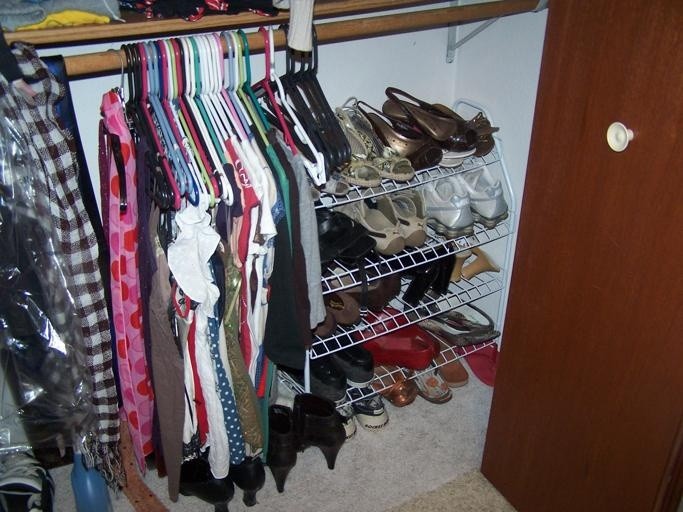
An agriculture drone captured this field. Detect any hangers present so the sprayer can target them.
[100,20,350,214]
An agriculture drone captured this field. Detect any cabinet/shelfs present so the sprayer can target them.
[277,100,527,412]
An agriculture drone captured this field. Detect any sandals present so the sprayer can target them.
[313,88,498,202]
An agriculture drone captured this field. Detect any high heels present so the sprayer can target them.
[180,394,346,512]
[279,250,499,340]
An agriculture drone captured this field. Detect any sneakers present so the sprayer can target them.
[304,297,500,440]
[314,162,508,270]
